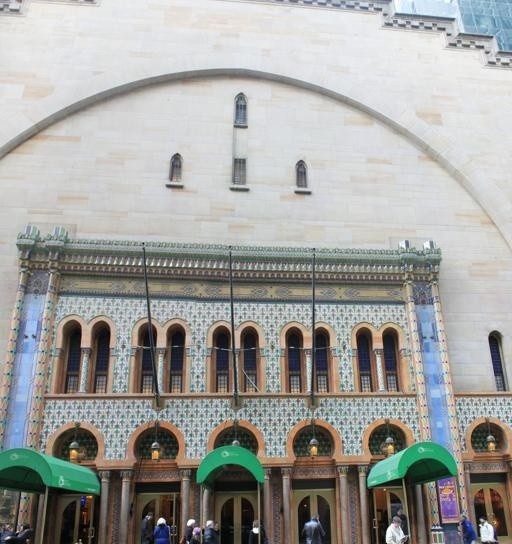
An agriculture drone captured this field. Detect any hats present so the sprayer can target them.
[157,518,166,526]
[187,519,213,534]
[393,517,401,522]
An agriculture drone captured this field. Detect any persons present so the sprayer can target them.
[140,512,155,543]
[459,514,498,544]
[153,517,169,544]
[0,520,30,544]
[183,518,219,544]
[249,520,266,544]
[386,516,409,544]
[301,513,326,544]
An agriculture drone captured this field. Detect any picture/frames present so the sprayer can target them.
[436,476,460,524]
